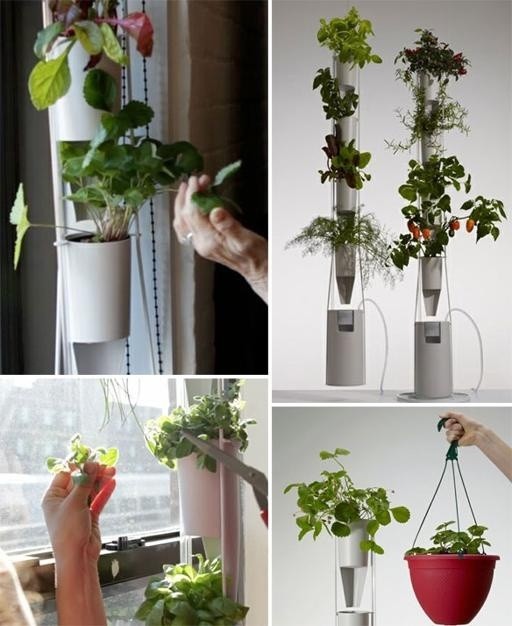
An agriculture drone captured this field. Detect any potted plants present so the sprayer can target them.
[284,448,410,569]
[144,379,257,539]
[9,101,244,345]
[28,0,154,143]
[404,520,500,626]
[283,6,508,316]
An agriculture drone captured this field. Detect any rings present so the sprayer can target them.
[185,230,198,243]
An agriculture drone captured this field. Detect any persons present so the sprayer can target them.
[41,452,116,626]
[172,173,266,308]
[436,411,512,482]
